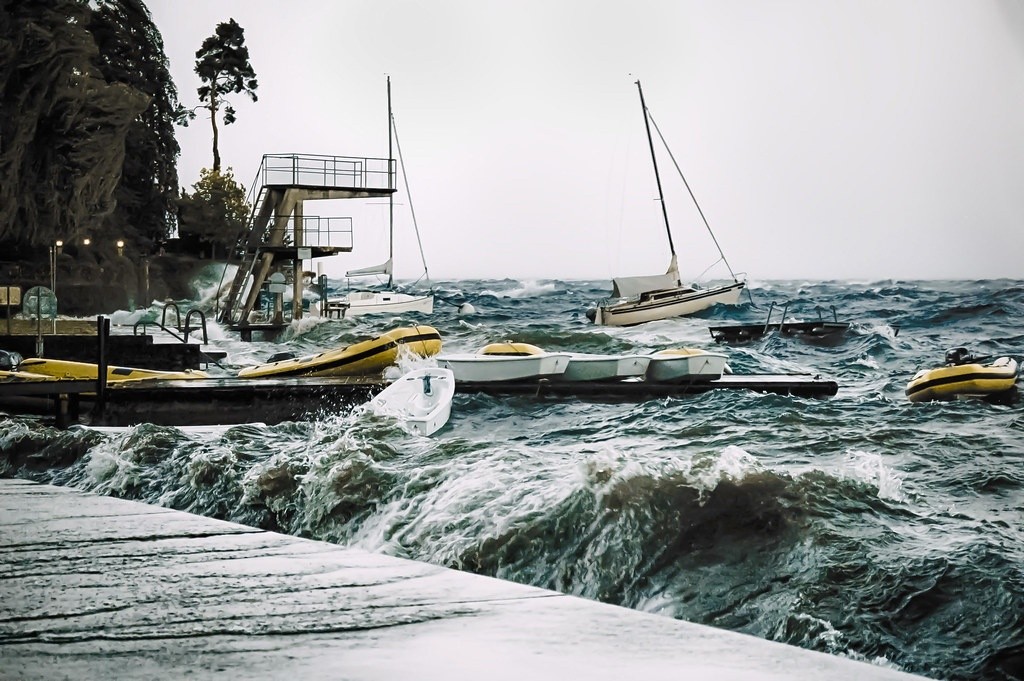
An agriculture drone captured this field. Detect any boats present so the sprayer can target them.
[0,350,58,383]
[19,358,212,382]
[707,299,900,346]
[644,346,729,382]
[239,320,444,381]
[476,342,546,356]
[434,348,573,383]
[905,346,1019,403]
[552,352,652,382]
[359,365,455,436]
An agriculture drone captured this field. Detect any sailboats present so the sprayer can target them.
[586,79,750,327]
[308,73,436,318]
